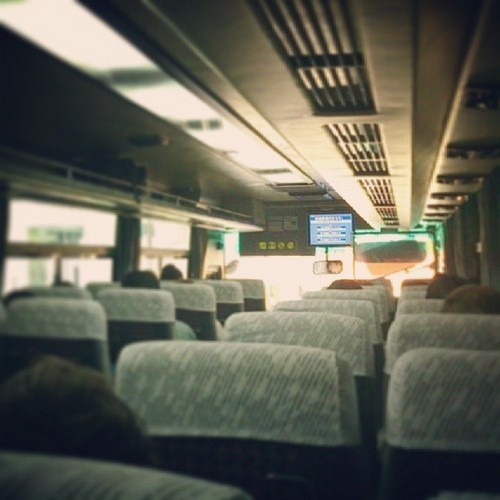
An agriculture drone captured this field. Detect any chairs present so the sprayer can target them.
[98,288,177,373]
[0,299,111,378]
[273,280,448,358]
[161,284,218,342]
[379,347,499,500]
[194,280,244,326]
[224,311,375,461]
[383,314,500,410]
[239,279,267,312]
[115,340,357,500]
[1,452,255,500]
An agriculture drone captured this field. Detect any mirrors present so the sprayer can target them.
[313,261,342,275]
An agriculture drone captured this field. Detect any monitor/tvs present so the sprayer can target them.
[308,212,355,248]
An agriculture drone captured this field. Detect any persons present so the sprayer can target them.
[0,352,145,468]
[328,271,500,316]
[0,265,237,351]
